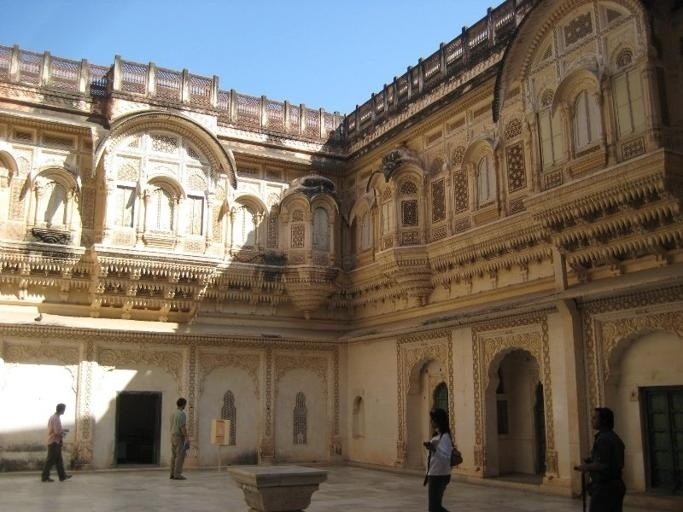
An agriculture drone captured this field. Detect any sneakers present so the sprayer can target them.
[169,475,186,480]
[42,473,73,483]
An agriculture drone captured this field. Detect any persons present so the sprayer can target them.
[423,407,453,512]
[169,398,190,480]
[41,403,73,482]
[574,407,626,512]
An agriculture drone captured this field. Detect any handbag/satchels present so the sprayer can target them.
[449,448,463,467]
